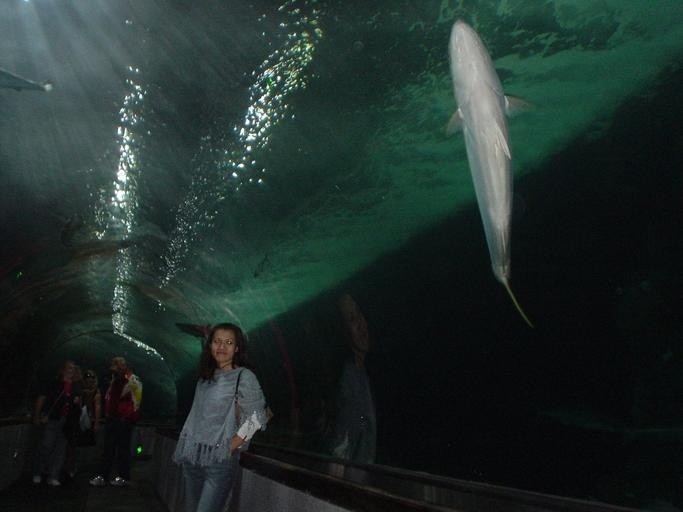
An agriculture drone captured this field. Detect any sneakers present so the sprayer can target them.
[32,472,129,487]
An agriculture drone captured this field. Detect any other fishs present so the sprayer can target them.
[442,19,538,328]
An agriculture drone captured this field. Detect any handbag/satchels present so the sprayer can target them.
[32,413,49,425]
[232,367,274,433]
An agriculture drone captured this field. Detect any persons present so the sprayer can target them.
[169,323,270,512]
[322,287,382,487]
[21,352,147,491]
